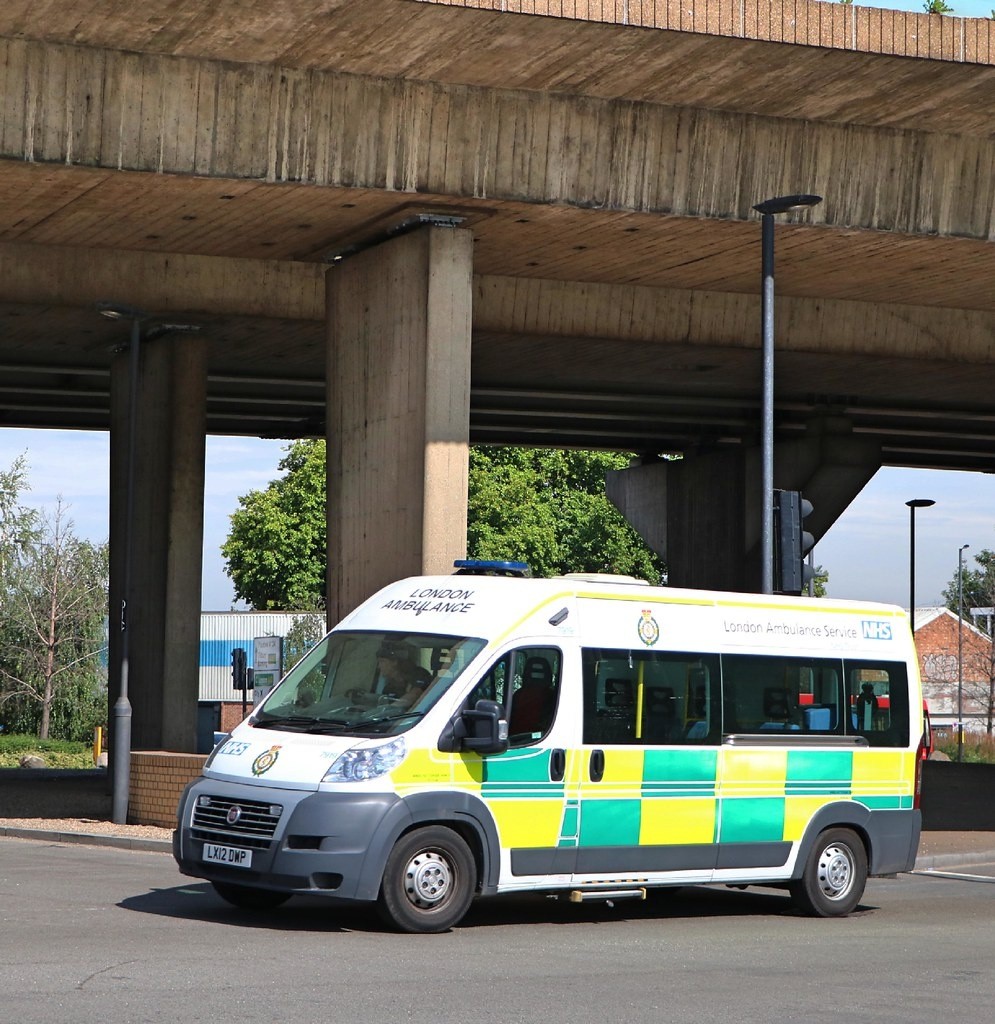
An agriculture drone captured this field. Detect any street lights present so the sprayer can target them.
[752,193,823,592]
[907,498,936,641]
[957,544,971,761]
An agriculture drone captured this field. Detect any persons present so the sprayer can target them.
[355,640,433,710]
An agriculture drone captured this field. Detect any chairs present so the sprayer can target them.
[758,687,800,731]
[599,678,637,739]
[646,685,681,740]
[430,648,457,688]
[510,655,556,737]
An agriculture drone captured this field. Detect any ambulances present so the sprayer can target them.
[171,559,928,935]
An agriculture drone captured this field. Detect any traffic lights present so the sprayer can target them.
[231,646,246,690]
[777,488,816,595]
